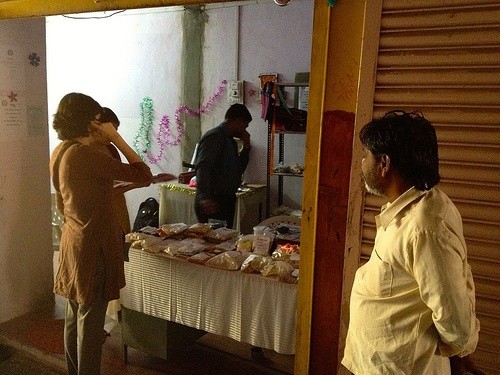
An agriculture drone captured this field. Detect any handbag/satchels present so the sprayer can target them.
[132,197,159,233]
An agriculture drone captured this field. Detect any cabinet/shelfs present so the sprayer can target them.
[265,81,310,219]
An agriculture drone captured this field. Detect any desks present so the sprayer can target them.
[120,245,298,365]
[158,182,267,232]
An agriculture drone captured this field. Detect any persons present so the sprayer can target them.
[341,110,480,375]
[49,92,153,375]
[194,103,252,229]
